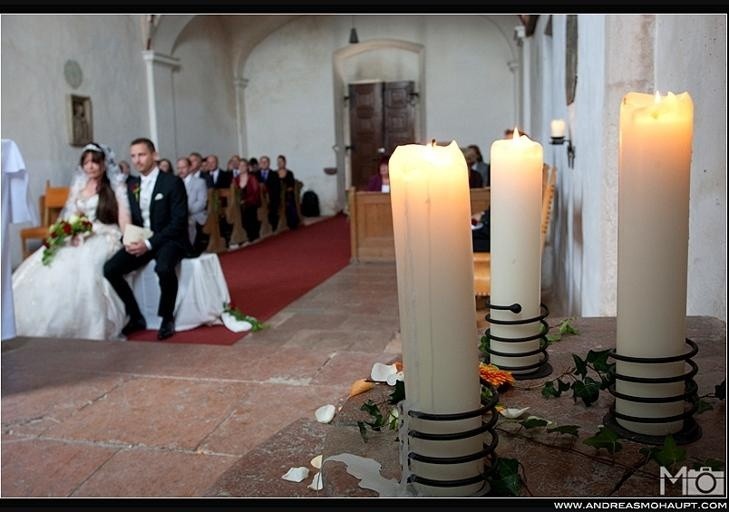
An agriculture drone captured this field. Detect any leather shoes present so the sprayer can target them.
[117,318,146,338]
[158,318,174,340]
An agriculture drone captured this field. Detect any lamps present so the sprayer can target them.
[343,15,365,45]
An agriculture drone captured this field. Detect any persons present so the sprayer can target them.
[461,146,485,188]
[101,137,200,342]
[469,144,490,187]
[362,152,392,193]
[116,149,302,254]
[469,201,491,253]
[11,139,135,341]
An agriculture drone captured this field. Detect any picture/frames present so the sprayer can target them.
[68,94,94,146]
[389,92,701,498]
